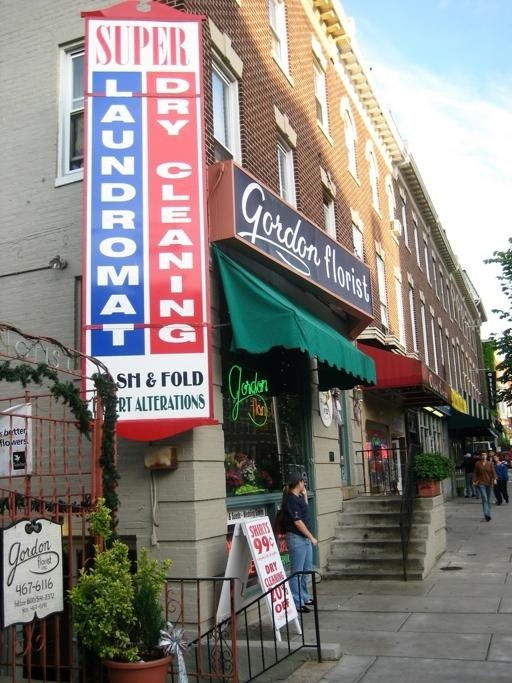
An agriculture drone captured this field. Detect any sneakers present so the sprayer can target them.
[304,597,314,605]
[296,605,311,613]
[463,493,476,498]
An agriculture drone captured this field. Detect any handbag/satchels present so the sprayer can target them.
[273,508,290,534]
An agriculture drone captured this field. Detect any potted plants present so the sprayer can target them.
[62,495,174,683]
[410,451,456,498]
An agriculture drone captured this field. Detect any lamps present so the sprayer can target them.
[49,254,67,270]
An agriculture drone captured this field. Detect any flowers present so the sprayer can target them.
[223,451,267,497]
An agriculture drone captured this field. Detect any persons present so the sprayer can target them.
[274,471,319,613]
[454,447,509,522]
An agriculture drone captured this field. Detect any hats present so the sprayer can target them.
[286,469,306,481]
